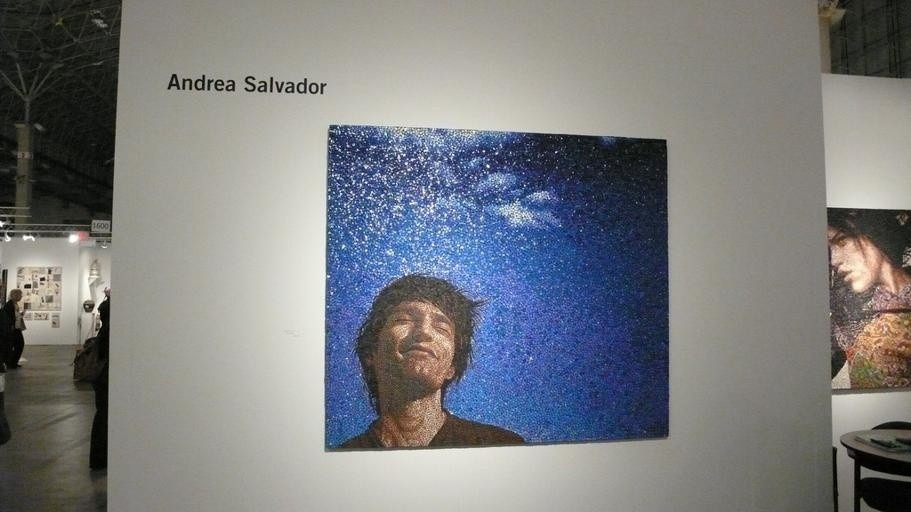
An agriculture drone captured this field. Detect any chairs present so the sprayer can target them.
[1,361,26,375]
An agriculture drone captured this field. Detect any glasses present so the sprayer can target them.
[0,210,108,249]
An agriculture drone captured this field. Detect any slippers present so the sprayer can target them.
[72,318,108,395]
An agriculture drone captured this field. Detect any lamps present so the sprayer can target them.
[853,422,911,511]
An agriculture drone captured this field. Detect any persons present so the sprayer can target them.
[829,209,911,392]
[90,306,110,472]
[4,290,27,370]
[325,272,528,452]
[0,303,13,446]
[98,286,110,311]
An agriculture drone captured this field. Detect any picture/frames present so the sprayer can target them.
[840,428,911,511]
